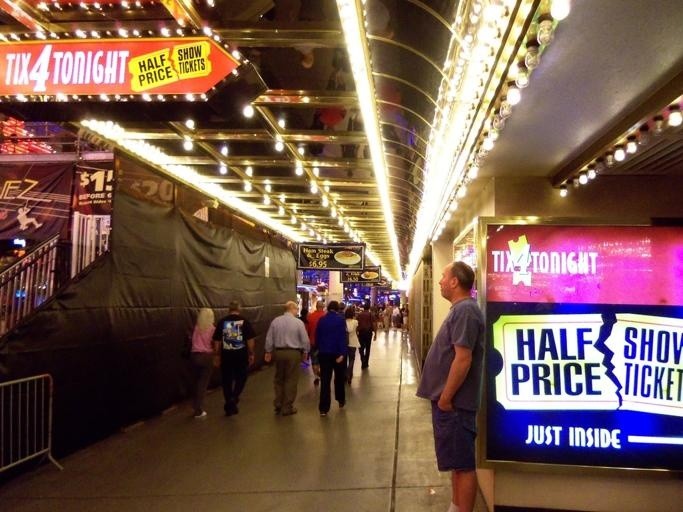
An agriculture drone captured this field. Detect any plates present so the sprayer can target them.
[361,271,379,279]
[334,251,362,265]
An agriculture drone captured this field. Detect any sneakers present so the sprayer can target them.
[320,411,326,417]
[193,410,207,418]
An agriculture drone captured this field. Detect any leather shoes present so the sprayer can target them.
[283,407,298,416]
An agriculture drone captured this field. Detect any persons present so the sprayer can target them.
[210,300,255,417]
[312,301,348,417]
[300,283,409,387]
[414,260,487,512]
[263,301,311,416]
[192,307,216,419]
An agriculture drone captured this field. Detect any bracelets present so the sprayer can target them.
[248,352,253,356]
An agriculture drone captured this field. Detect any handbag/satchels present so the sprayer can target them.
[180,320,196,361]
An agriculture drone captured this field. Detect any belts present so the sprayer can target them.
[276,348,300,350]
